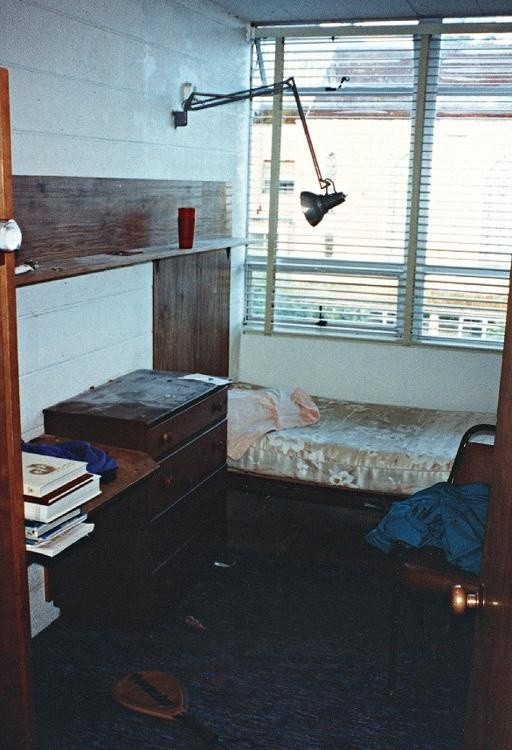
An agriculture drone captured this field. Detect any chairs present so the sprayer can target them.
[387,423,496,710]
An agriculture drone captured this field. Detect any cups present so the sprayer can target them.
[177,207,196,249]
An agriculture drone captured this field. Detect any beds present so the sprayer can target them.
[229,380,498,514]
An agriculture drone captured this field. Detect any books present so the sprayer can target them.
[21,451,103,559]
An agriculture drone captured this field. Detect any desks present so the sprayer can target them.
[22,434,161,629]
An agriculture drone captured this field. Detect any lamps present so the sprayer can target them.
[175,75,346,226]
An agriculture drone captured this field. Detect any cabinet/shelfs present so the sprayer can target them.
[43,369,231,630]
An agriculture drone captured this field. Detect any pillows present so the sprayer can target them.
[226,385,321,462]
